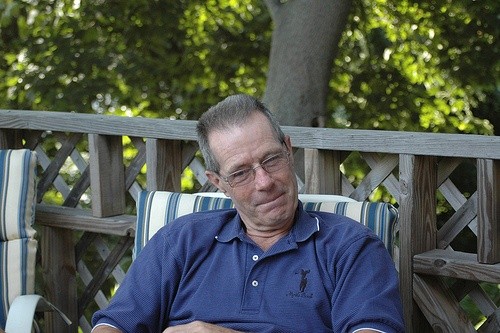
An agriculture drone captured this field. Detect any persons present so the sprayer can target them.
[90,93,405,333]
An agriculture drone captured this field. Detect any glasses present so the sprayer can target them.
[214,141,291,188]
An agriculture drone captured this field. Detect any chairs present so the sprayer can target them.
[136,190,399,333]
[0,148,55,333]
